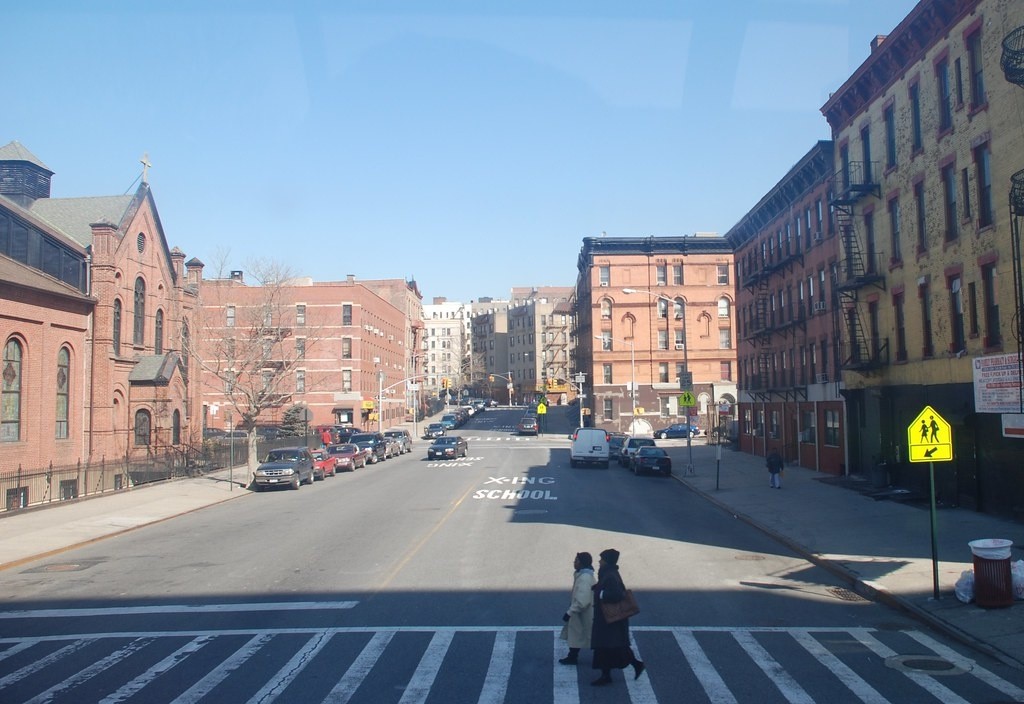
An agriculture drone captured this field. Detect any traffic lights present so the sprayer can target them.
[441,378,451,389]
[548,378,557,390]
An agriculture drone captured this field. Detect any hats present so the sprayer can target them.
[577,552,592,567]
[599,549,620,566]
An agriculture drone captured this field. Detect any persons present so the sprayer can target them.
[767,448,783,489]
[559,552,596,664]
[321,430,331,448]
[590,549,646,685]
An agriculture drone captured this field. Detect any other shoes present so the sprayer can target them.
[558,653,577,665]
[771,486,773,488]
[634,662,645,680]
[776,487,780,489]
[591,673,612,686]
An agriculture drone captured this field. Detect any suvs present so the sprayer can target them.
[255,446,315,492]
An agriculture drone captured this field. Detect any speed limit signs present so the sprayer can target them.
[680,373,693,391]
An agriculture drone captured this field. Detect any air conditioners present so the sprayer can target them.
[676,344,684,349]
[816,373,829,383]
[814,302,825,311]
[813,232,822,241]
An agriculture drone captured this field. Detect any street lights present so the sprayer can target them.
[413,348,445,438]
[593,335,635,439]
[622,289,696,477]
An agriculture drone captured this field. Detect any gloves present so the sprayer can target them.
[563,613,570,621]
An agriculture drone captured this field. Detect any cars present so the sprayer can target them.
[427,436,468,460]
[308,425,412,480]
[424,423,448,439]
[439,398,499,430]
[654,422,700,439]
[518,403,541,436]
[567,427,671,477]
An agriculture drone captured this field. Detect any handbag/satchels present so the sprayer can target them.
[780,470,784,476]
[600,572,640,624]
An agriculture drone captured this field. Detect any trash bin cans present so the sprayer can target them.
[967,538,1014,609]
